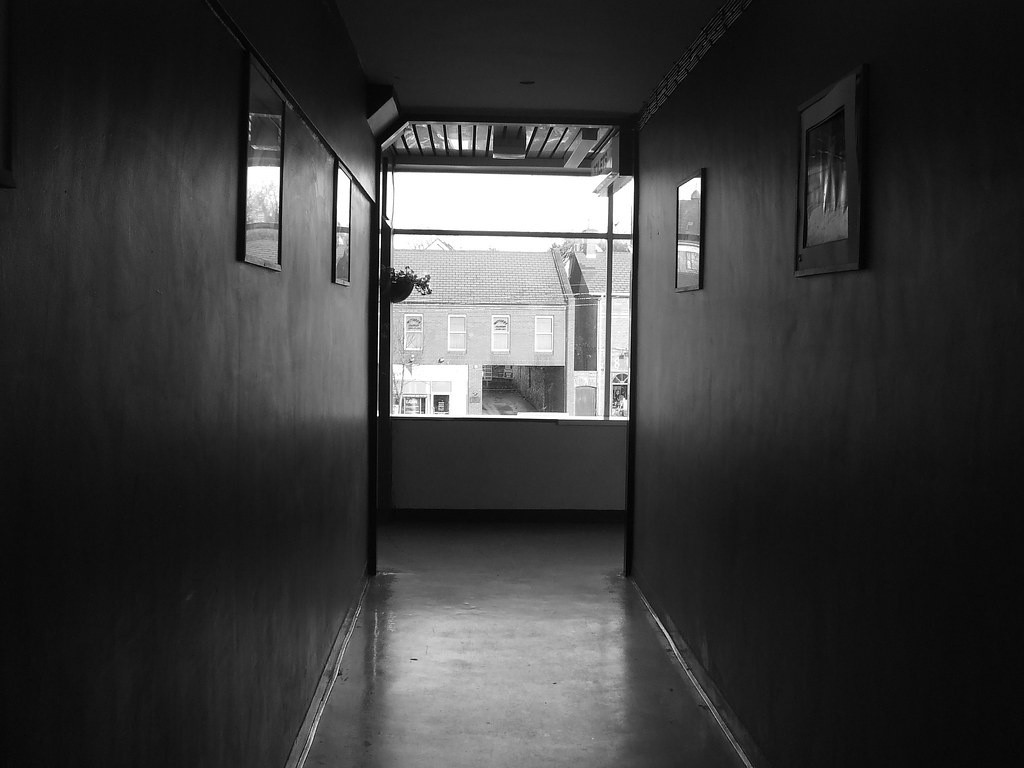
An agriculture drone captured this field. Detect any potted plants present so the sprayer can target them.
[381,265,432,304]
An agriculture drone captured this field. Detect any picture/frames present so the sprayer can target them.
[331,160,353,288]
[237,52,290,273]
[789,61,870,278]
[672,166,708,294]
